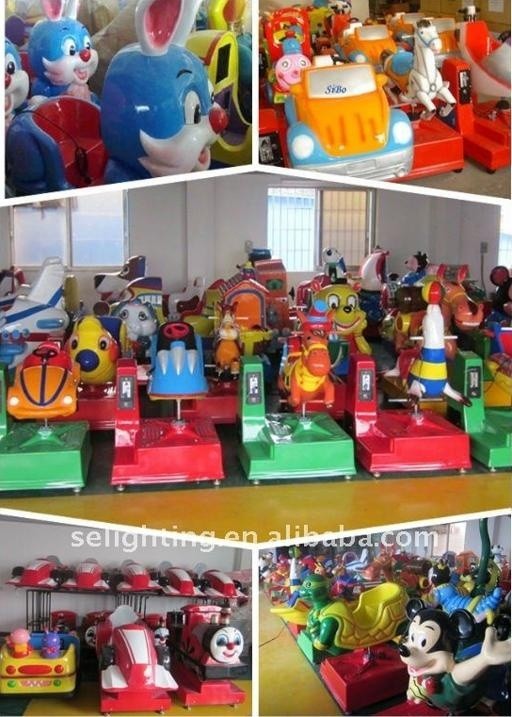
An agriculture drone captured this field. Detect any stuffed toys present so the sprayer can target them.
[259,542,511,716]
[0,555,253,713]
[259,0,511,184]
[5,0,253,200]
[0,249,512,491]
